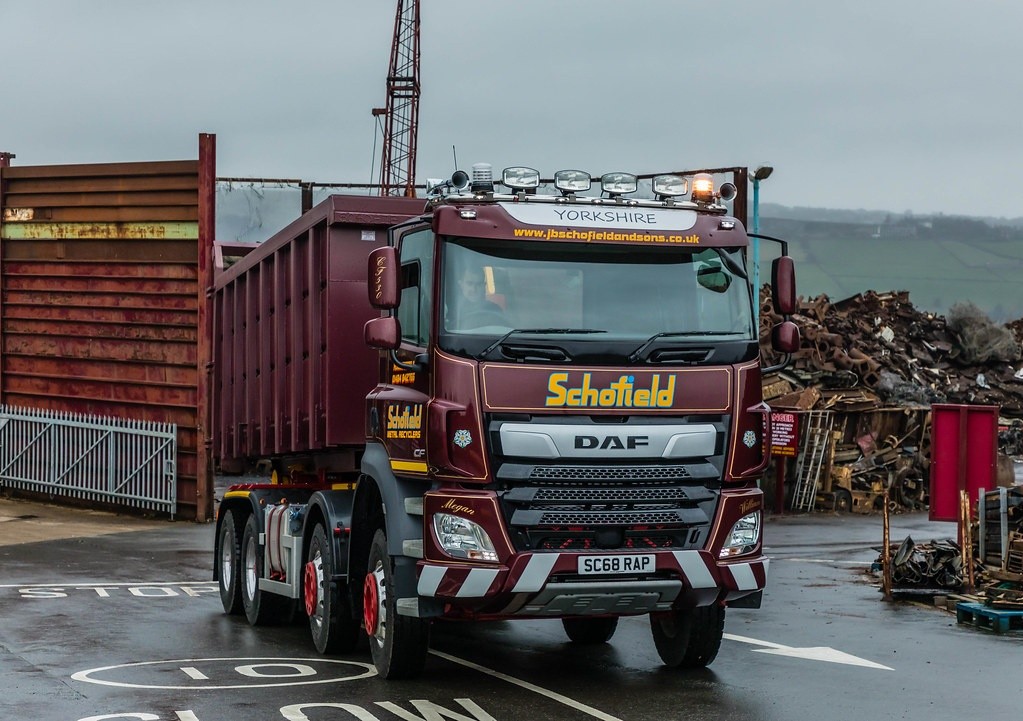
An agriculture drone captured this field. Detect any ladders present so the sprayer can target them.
[791,410,834,512]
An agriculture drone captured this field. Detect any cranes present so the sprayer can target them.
[369,0,426,199]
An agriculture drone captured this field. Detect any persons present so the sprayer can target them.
[444,262,505,330]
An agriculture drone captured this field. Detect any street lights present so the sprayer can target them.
[748,165,775,342]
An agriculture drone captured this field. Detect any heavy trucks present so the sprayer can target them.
[203,164,802,682]
